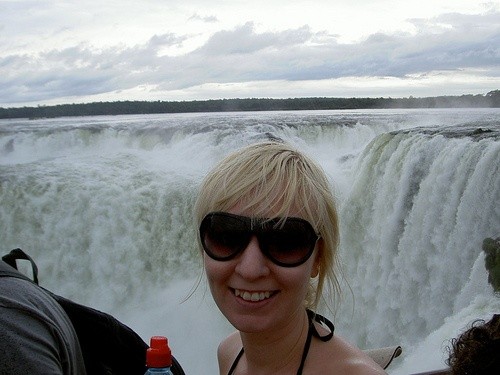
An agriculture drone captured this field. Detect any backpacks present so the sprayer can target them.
[0,248,186,375]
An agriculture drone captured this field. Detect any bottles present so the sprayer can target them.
[143,337,174,375]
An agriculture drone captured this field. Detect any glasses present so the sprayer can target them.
[199,211,321,267]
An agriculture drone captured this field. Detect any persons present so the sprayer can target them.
[194,141,389,375]
[0,258,88,375]
